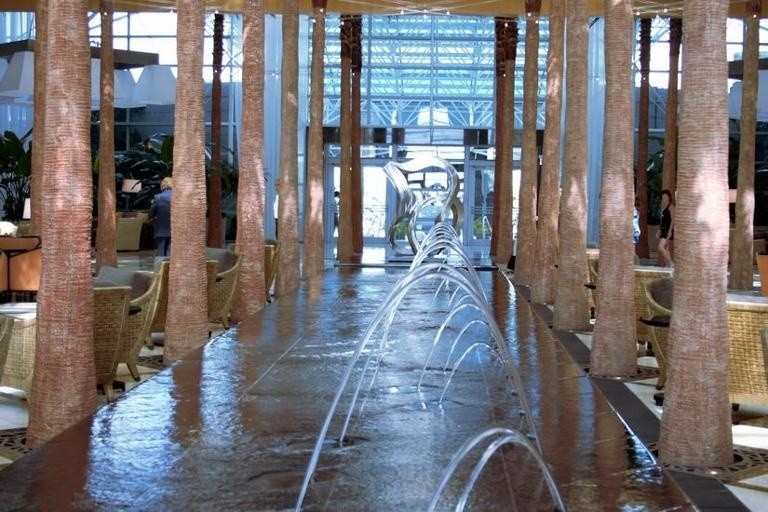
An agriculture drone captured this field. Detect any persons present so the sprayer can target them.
[148,176,173,255]
[273,178,280,241]
[655,189,676,267]
[633,188,642,267]
[334,191,340,233]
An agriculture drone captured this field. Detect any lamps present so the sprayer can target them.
[122,179,142,210]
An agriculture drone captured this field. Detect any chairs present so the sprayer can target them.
[90,285,134,404]
[1,312,37,405]
[113,261,169,380]
[588,224,768,422]
[264,237,281,255]
[206,250,243,332]
[132,259,220,350]
[229,242,275,305]
[2,211,152,302]
